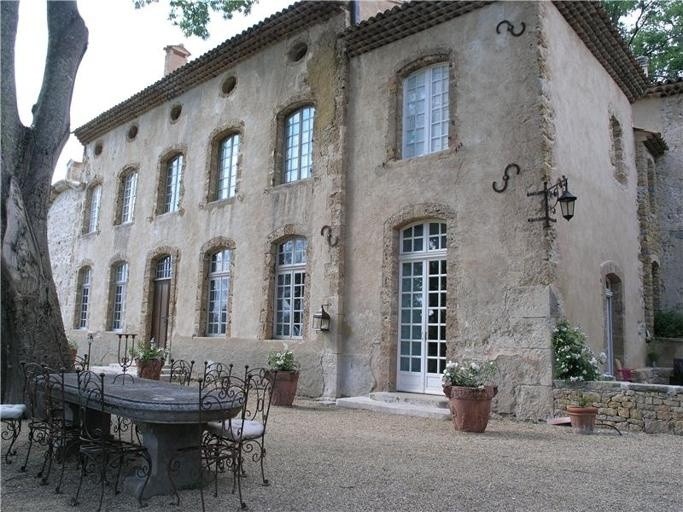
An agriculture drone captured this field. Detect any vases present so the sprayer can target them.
[266,368,299,407]
[445,385,499,432]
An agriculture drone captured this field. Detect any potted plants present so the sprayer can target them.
[567,394,599,435]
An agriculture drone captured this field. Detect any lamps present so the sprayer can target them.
[311,303,332,331]
[557,179,576,221]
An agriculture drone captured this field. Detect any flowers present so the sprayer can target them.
[443,358,498,392]
[128,338,164,360]
[266,350,298,371]
